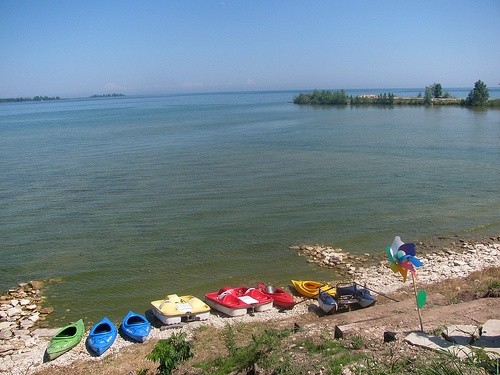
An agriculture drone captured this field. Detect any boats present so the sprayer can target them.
[121,310,151,342]
[46,318,85,360]
[204,286,273,316]
[87,316,117,355]
[256,280,297,309]
[316,281,378,314]
[290,279,337,299]
[150,294,211,325]
